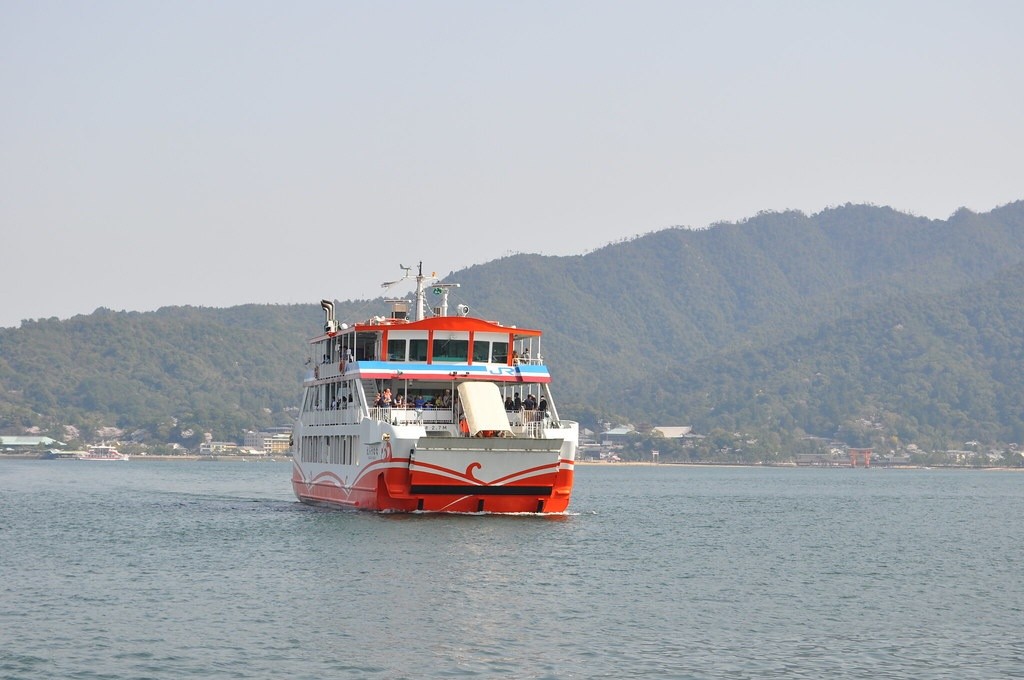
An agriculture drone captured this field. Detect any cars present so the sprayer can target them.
[286,452,294,457]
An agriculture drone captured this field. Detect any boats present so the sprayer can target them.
[288,261,578,513]
[80,440,130,461]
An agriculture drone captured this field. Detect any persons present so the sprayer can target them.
[315,395,357,409]
[374,389,552,438]
[508,346,534,367]
[321,346,357,363]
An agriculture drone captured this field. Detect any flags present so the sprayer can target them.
[305,357,312,365]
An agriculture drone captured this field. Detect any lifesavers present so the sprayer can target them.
[313,366,318,378]
[339,360,344,374]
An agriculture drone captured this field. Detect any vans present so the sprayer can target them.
[200,448,213,455]
